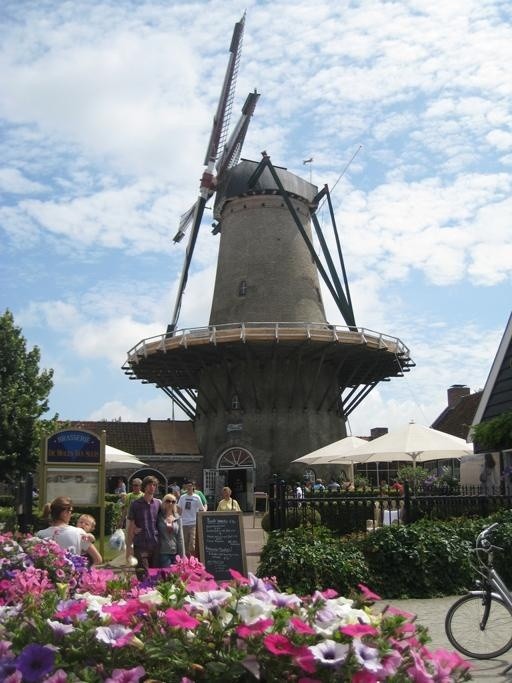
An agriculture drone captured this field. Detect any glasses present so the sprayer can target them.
[63,508,74,515]
[164,499,177,505]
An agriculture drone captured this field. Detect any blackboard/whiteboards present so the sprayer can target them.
[197,511,248,585]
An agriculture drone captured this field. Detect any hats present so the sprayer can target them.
[133,478,142,486]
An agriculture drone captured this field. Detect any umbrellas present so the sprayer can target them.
[328,419,477,479]
[291,434,372,474]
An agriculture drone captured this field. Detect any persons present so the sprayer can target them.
[216,486,241,510]
[134,493,186,567]
[177,480,207,558]
[34,496,104,567]
[126,475,182,567]
[479,453,497,486]
[77,513,96,560]
[119,477,209,529]
[290,478,355,500]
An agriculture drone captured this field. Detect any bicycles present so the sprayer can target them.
[445,522,511,676]
